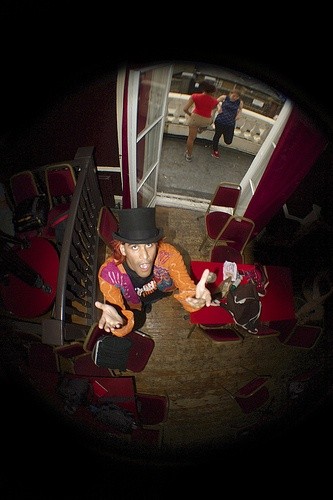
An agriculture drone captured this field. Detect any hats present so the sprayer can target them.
[111,208,163,245]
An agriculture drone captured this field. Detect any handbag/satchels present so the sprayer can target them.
[57,378,94,407]
[222,283,262,335]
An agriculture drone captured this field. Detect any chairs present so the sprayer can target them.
[250,327,279,338]
[44,164,77,229]
[9,170,40,232]
[132,394,172,450]
[96,206,124,247]
[80,322,156,373]
[54,342,103,377]
[207,215,256,264]
[197,326,243,345]
[217,364,273,417]
[197,181,243,252]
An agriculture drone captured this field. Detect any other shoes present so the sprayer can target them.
[144,304,151,313]
[212,150,219,158]
[185,152,192,161]
[198,128,203,133]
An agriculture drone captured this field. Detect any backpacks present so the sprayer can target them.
[91,335,130,372]
[11,193,48,231]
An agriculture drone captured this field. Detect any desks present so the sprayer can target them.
[2,238,62,317]
[89,377,135,421]
[188,260,296,340]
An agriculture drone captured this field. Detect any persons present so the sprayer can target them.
[94,207,212,333]
[183,79,219,162]
[210,88,244,159]
